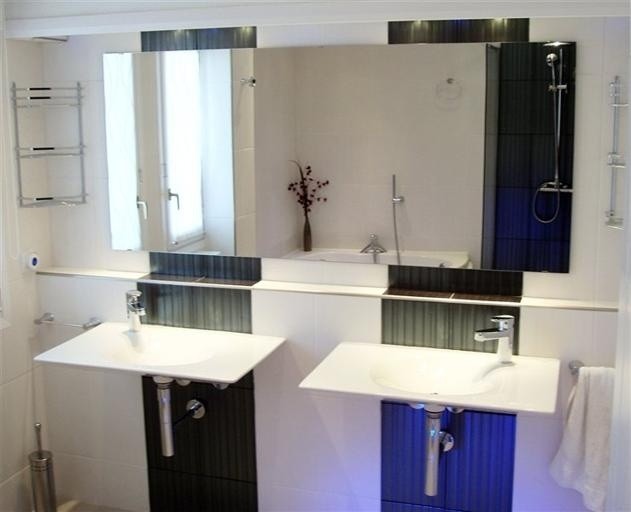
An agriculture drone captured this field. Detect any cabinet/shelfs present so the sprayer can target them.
[10,81,89,210]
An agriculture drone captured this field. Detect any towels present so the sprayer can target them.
[554,365,615,511]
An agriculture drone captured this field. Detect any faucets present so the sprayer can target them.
[362,234,386,256]
[475,315,514,361]
[125,288,144,332]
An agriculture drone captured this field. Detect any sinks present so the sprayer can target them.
[33,322,284,384]
[298,341,560,416]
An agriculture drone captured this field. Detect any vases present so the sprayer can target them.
[299,217,318,253]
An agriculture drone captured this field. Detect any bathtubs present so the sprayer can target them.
[283,246,469,269]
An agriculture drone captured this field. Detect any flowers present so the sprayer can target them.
[287,158,329,216]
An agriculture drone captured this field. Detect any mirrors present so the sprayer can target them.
[104,48,241,258]
[254,42,577,274]
